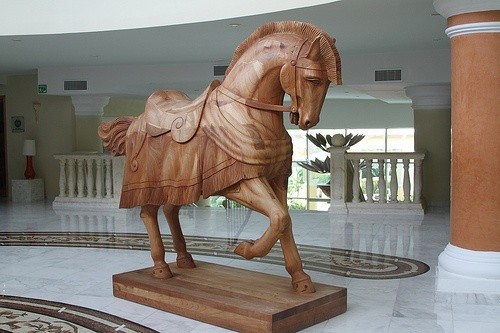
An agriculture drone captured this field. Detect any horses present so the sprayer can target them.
[96,20,343,294]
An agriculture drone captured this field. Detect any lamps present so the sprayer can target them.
[19,138,38,179]
